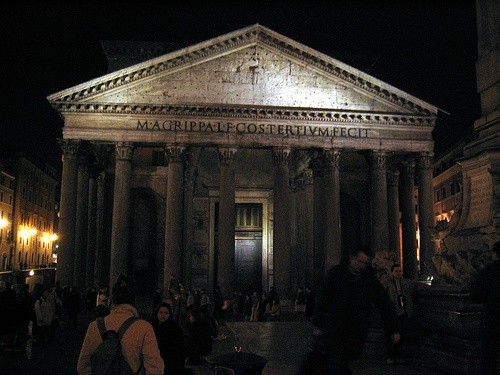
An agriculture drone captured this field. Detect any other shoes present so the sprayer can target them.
[386,358,395,365]
[395,359,405,363]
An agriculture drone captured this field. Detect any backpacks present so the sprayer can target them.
[90,317,146,375]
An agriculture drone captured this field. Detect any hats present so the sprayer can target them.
[112,272,134,300]
[99,278,108,288]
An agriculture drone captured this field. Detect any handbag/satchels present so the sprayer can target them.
[297,335,333,375]
[40,302,53,322]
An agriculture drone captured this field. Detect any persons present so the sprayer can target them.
[294,240,500,375]
[0,260,281,375]
